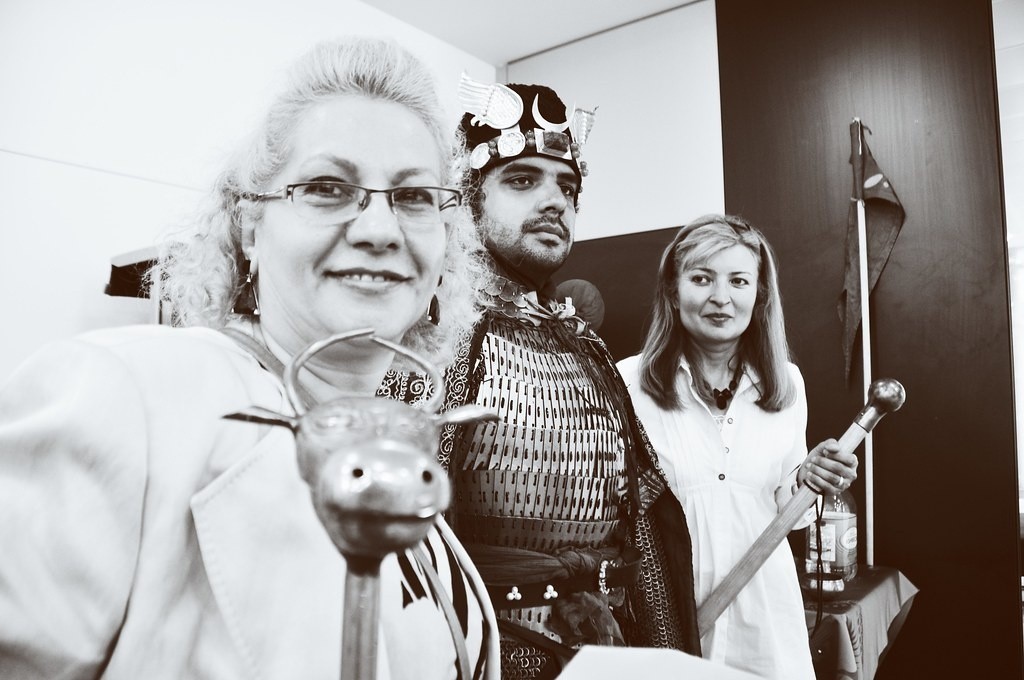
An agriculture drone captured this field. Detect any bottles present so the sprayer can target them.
[810,488,859,583]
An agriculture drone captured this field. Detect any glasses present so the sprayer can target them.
[251,181,462,223]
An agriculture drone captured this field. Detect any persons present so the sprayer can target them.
[613,215,858,680]
[0,35,702,680]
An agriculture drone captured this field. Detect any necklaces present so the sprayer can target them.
[713,360,742,410]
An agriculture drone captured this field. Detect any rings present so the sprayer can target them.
[834,477,843,487]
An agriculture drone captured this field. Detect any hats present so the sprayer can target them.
[466,82,598,207]
